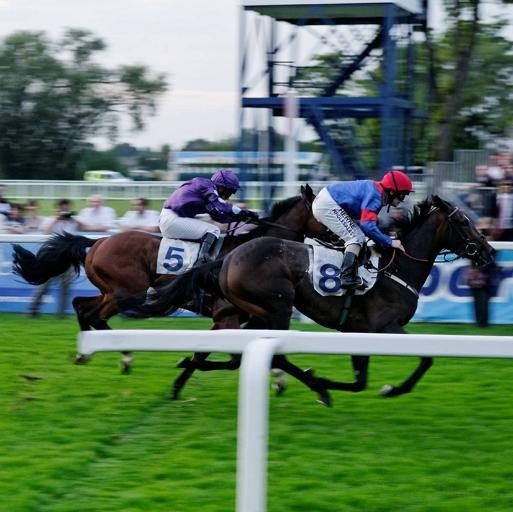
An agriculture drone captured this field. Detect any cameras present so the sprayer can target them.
[56,209,79,220]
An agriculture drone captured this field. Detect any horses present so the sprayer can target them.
[12,183,345,396]
[113,194,498,408]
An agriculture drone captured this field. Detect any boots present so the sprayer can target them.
[197,232,218,265]
[339,251,369,290]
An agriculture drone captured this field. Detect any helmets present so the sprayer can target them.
[380,170,416,193]
[210,169,241,191]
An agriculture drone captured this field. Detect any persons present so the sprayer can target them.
[0,184,159,234]
[458,150,513,240]
[160,170,258,268]
[312,170,415,287]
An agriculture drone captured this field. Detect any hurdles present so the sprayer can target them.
[76,331,513,512]
